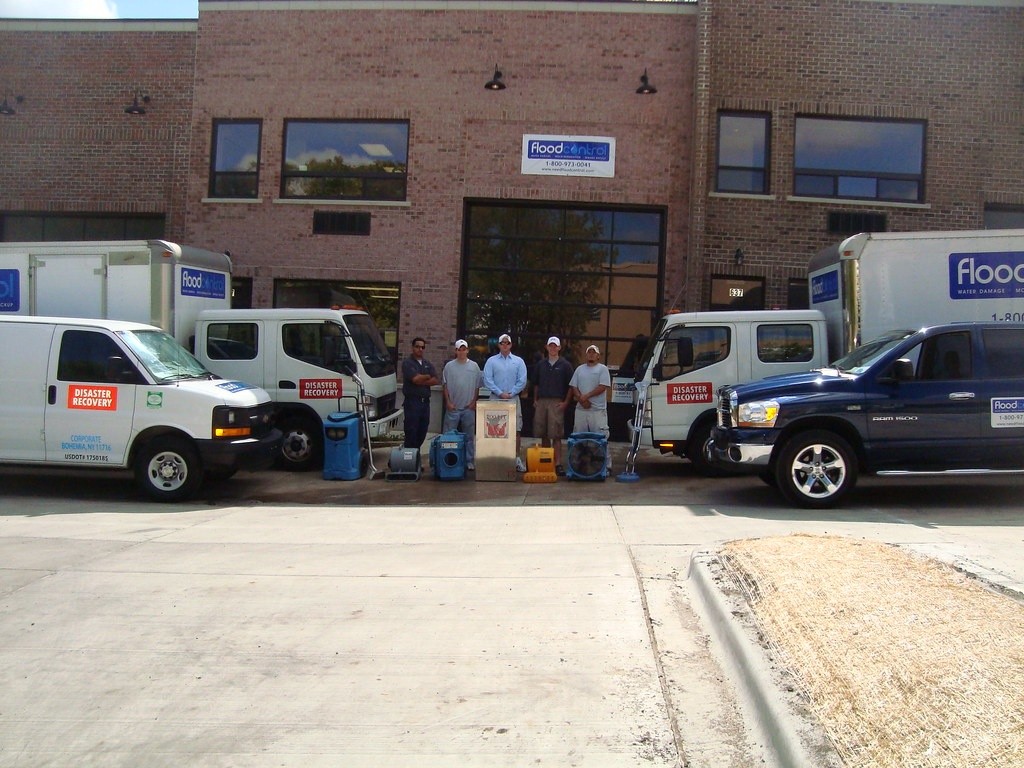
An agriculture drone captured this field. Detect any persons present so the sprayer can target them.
[401,338,439,471]
[568,345,613,477]
[483,334,527,473]
[531,336,575,475]
[442,339,482,469]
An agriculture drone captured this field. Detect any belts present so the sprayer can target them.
[406,394,429,402]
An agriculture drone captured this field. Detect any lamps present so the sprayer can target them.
[124,88,150,115]
[484,63,506,90]
[635,67,658,94]
[734,248,745,267]
[0,87,23,115]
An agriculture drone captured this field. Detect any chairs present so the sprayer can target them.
[942,351,961,378]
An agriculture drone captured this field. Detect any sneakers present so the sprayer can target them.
[468,463,475,470]
[515,460,527,472]
[556,464,565,475]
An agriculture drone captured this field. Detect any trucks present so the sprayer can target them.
[0,239,404,471]
[0,314,276,504]
[631,227,1024,475]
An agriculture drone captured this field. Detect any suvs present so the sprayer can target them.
[713,319,1024,510]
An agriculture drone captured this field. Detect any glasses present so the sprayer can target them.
[499,341,510,345]
[414,345,425,350]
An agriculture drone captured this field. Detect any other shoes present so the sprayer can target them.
[606,470,610,475]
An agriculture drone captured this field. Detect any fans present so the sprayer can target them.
[566,432,608,481]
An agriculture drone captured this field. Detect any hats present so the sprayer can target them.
[499,334,511,344]
[586,345,599,354]
[547,336,561,347]
[455,339,469,349]
